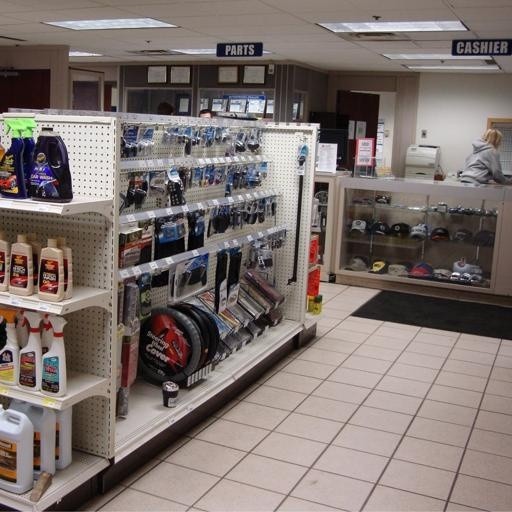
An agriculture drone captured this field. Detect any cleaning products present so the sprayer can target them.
[1,303,67,397]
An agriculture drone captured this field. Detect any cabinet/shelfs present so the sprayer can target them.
[338,177,512,308]
[0,106,340,512]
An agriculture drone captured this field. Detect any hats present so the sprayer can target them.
[344,255,433,279]
[350,219,495,248]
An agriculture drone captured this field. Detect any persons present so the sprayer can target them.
[158,102,176,116]
[456,128,511,187]
[199,109,213,118]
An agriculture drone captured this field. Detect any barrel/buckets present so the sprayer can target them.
[8,399,57,479]
[56,407,74,471]
[0,404,35,495]
